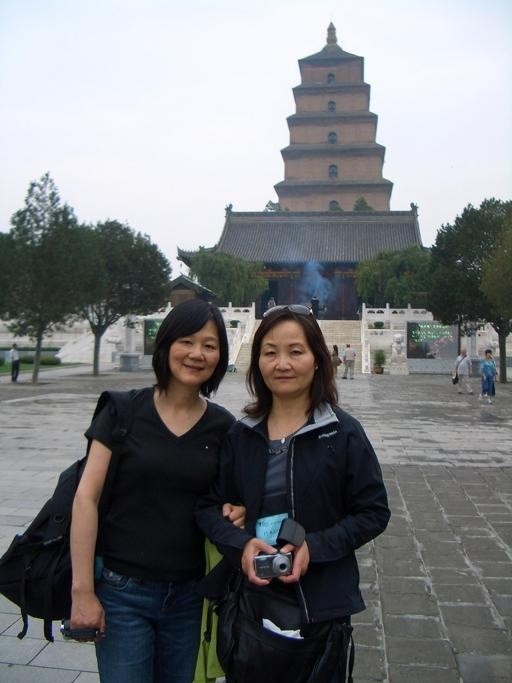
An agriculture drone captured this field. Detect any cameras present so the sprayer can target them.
[60,619,99,642]
[253,552,293,579]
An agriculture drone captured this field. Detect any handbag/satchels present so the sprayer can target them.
[204,581,354,682]
[452,373,457,384]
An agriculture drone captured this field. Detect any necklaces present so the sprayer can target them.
[270,416,304,444]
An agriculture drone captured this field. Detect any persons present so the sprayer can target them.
[453,347,473,394]
[9,343,20,382]
[193,304,390,683]
[332,344,338,377]
[311,296,319,318]
[480,350,497,404]
[70,298,237,683]
[342,344,358,379]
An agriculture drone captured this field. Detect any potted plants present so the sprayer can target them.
[373,349,385,374]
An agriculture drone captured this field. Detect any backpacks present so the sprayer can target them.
[0,390,132,624]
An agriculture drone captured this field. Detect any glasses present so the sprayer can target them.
[261,303,314,320]
